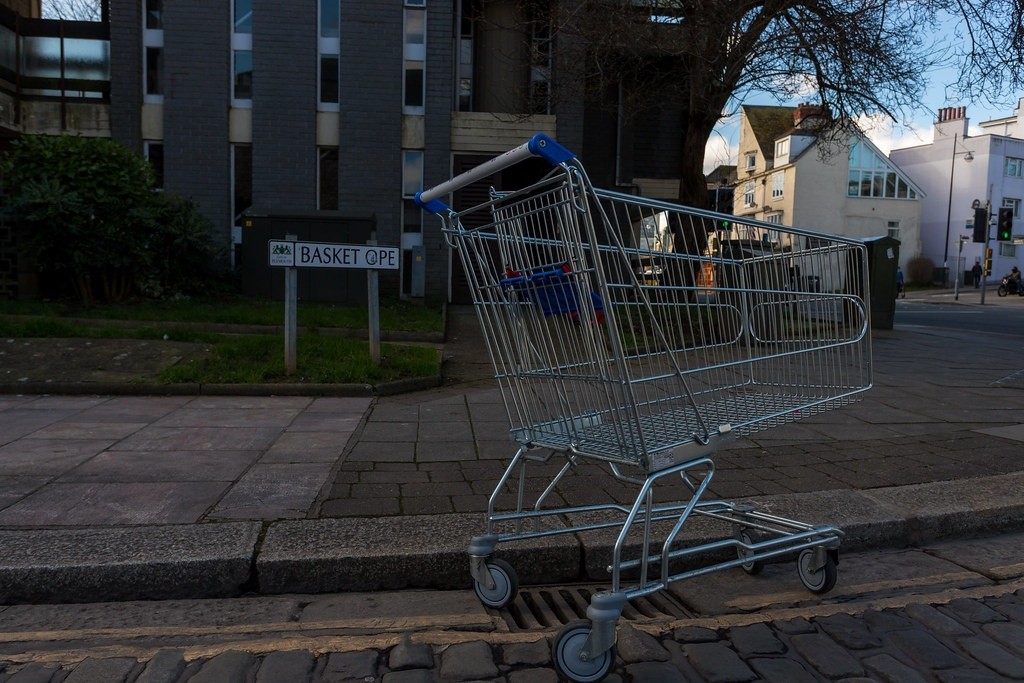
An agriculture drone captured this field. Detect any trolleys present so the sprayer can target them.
[416,132,872,683]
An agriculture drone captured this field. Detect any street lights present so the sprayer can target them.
[942,131,975,267]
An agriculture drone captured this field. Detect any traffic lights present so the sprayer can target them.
[997,207,1013,241]
[985,247,993,276]
[718,205,733,229]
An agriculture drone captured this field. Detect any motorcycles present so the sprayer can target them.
[998,273,1024,296]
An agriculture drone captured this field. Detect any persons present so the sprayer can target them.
[1008,266,1021,290]
[895,266,903,299]
[972,262,982,289]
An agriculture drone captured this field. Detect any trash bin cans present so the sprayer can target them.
[931,267,950,287]
[719,232,789,346]
[842,236,901,331]
[963,270,974,287]
